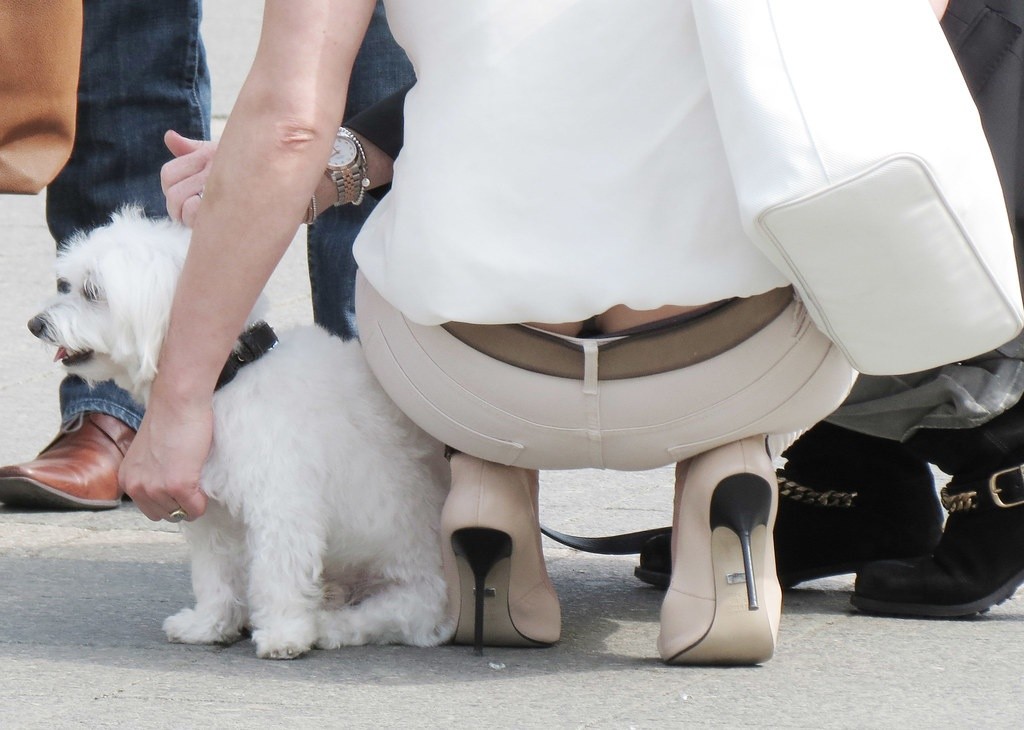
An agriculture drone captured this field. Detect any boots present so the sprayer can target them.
[850,396,1024,619]
[634,419,944,591]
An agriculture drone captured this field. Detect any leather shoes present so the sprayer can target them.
[0,409,139,510]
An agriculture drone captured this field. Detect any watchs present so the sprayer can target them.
[322,134,360,206]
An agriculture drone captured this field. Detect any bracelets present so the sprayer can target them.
[338,127,370,205]
[306,195,317,226]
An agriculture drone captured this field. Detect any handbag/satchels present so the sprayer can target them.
[691,0,1024,376]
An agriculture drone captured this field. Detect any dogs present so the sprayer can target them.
[27,198,453,660]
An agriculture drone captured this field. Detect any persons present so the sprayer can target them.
[116,0,948,665]
[0,0,418,511]
[161,0,1024,619]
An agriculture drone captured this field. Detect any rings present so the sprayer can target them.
[198,192,203,198]
[169,508,186,520]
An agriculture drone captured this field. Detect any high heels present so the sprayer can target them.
[658,435,782,665]
[439,452,561,657]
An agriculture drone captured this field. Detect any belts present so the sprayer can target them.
[440,283,795,380]
